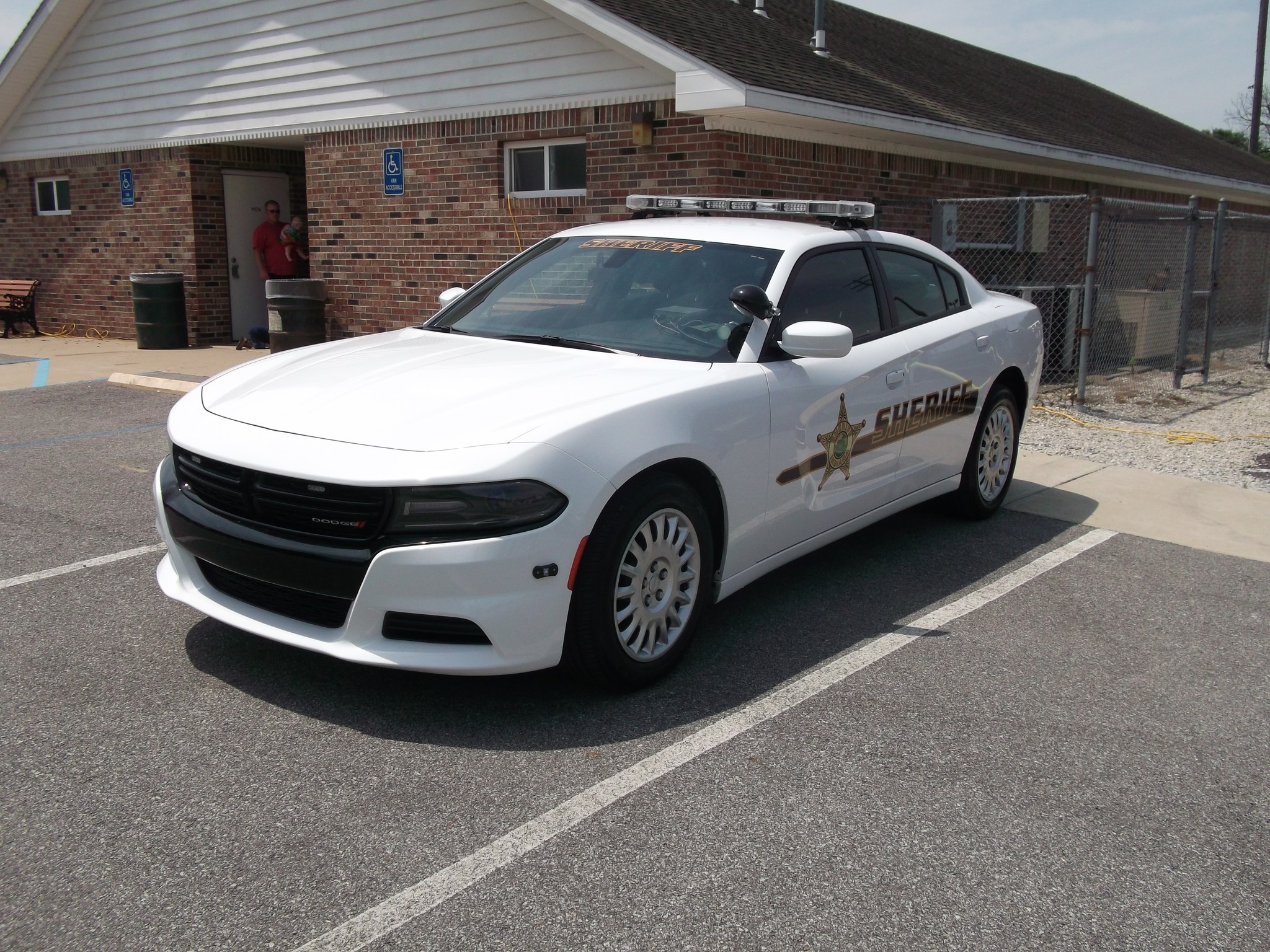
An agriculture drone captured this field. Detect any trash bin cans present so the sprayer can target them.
[129,272,188,349]
[265,279,328,354]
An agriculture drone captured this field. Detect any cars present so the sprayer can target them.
[151,198,1042,699]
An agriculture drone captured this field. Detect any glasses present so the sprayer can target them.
[265,209,280,214]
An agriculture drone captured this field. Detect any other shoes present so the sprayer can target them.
[236,336,247,350]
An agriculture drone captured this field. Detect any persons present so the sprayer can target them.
[253,200,299,282]
[235,326,270,350]
[279,217,310,261]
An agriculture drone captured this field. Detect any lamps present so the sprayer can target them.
[632,112,651,145]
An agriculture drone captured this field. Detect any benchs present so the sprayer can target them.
[0,280,42,337]
